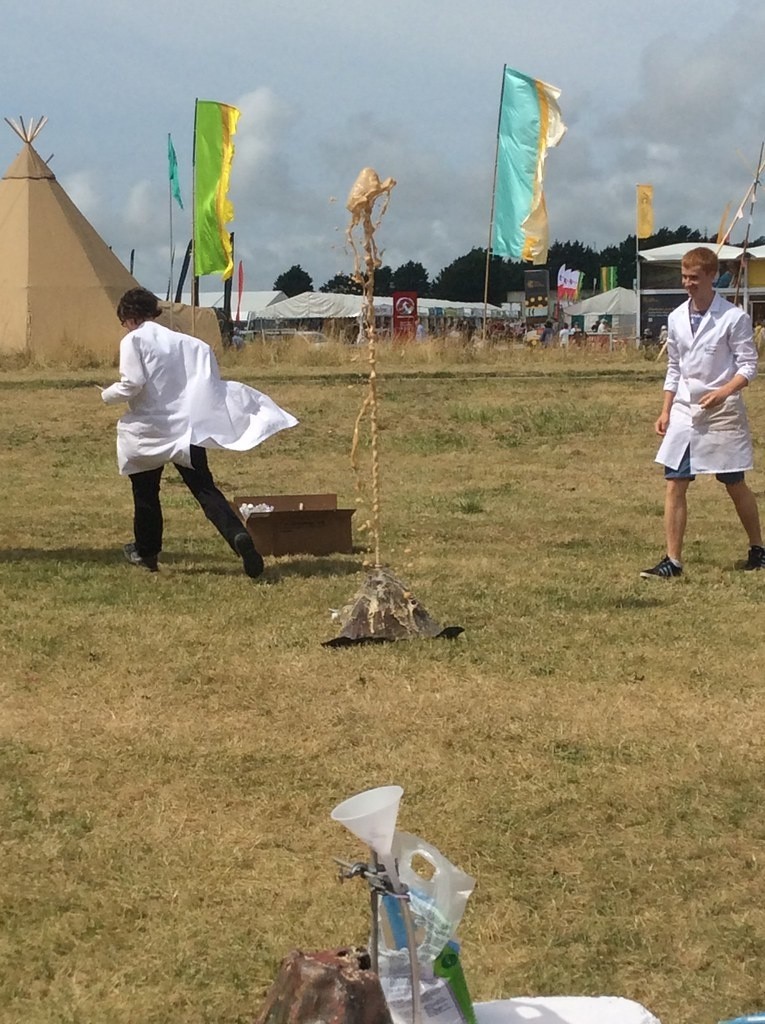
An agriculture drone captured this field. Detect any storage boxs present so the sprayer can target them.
[227,492,357,558]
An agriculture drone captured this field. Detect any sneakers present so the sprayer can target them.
[234,532,264,577]
[640,554,682,578]
[744,545,765,571]
[123,544,158,571]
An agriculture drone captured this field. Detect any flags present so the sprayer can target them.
[191,98,241,282]
[488,66,568,267]
[166,132,185,211]
[636,184,656,241]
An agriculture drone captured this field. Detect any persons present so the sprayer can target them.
[98,285,266,580]
[227,260,765,357]
[638,246,765,581]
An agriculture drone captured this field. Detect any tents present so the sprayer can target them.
[0,112,226,372]
